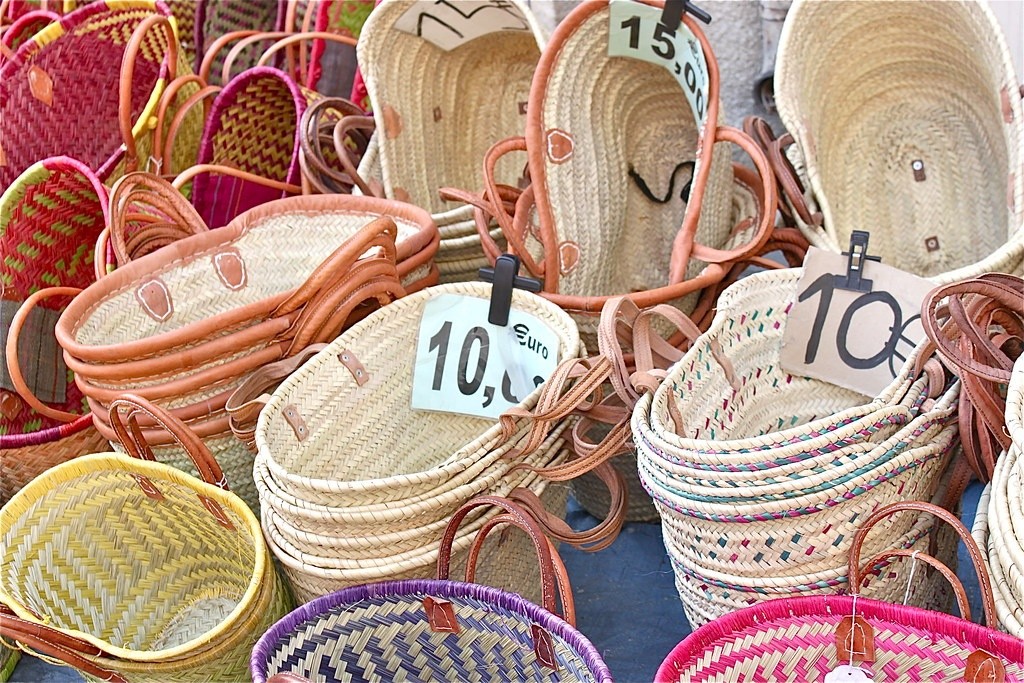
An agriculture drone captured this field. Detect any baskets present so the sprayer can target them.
[0,0,1024,683]
